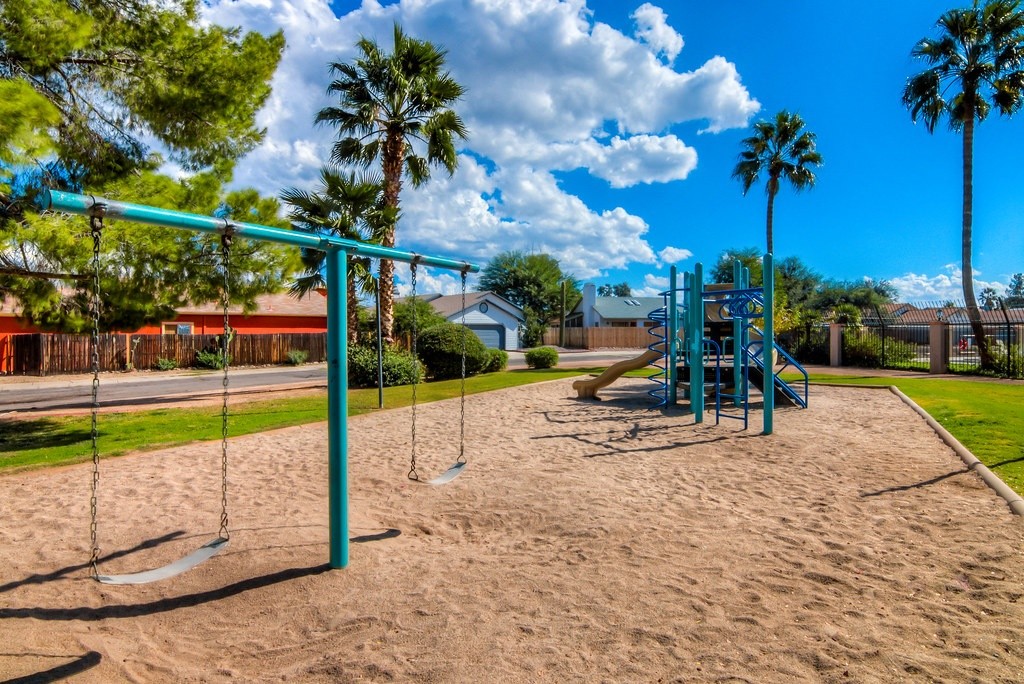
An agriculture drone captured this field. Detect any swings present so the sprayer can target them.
[87,214,231,585]
[408,263,468,486]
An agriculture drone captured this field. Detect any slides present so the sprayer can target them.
[572,341,679,390]
[705,281,778,396]
